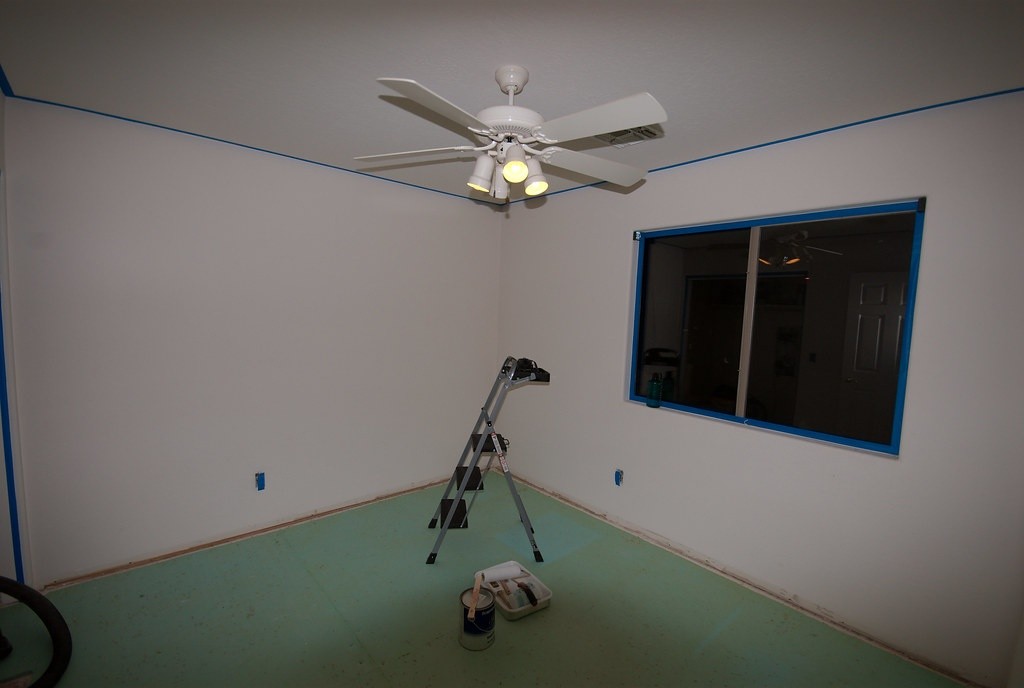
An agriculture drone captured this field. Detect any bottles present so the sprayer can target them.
[647,373,662,408]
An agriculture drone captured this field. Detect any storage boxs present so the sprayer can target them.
[474,560,552,621]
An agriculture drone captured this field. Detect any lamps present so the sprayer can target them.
[466,153,495,193]
[523,157,549,195]
[489,165,510,199]
[502,144,529,184]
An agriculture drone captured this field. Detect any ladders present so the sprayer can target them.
[425,354,551,565]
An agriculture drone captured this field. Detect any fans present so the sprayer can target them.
[352,65,668,187]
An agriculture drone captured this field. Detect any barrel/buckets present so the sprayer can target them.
[459,588,496,651]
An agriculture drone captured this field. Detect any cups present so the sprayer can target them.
[510,583,543,609]
